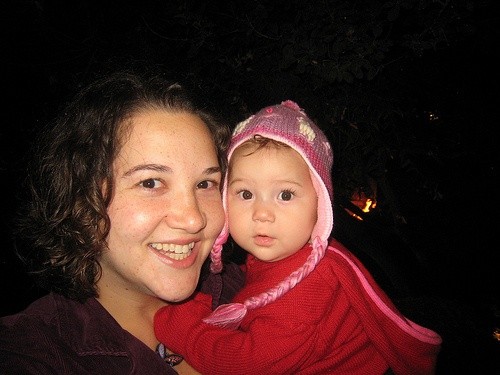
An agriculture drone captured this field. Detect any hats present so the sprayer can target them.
[202,100,333,328]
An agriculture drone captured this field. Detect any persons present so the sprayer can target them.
[0,60,250,375]
[138,99,443,375]
